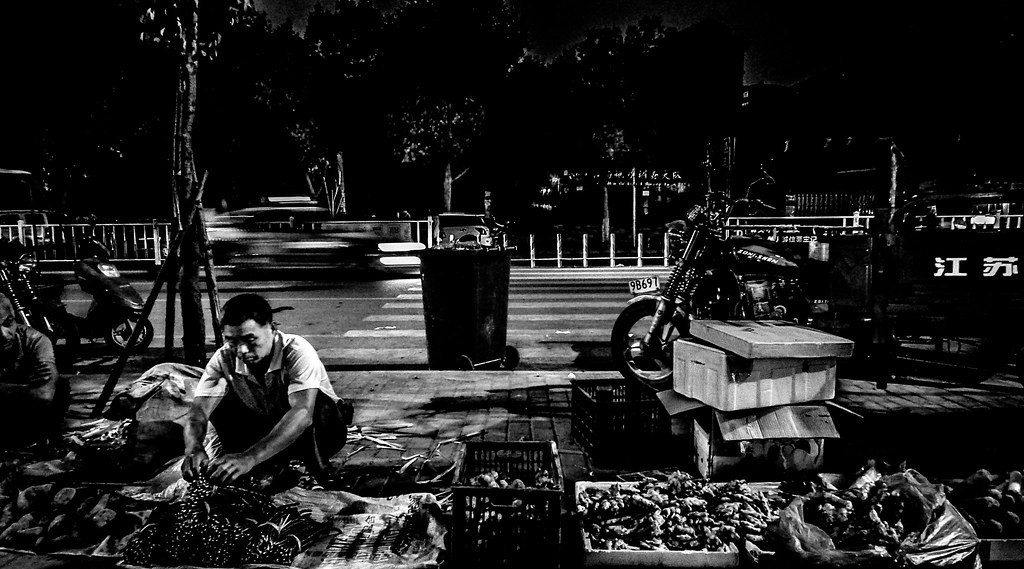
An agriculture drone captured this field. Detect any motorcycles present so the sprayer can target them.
[611,139,800,393]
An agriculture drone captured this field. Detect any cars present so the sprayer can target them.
[207,201,387,282]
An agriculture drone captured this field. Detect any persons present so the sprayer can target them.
[181,295,354,496]
[0,293,71,463]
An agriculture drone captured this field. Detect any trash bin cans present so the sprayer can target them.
[412,244,521,372]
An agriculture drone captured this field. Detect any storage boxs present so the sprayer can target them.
[671,320,855,412]
[449,440,564,569]
[656,389,865,483]
[568,378,673,470]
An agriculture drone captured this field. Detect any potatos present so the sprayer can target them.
[948,469,1024,533]
[0,482,123,552]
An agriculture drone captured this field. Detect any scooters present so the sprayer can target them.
[0,212,156,356]
